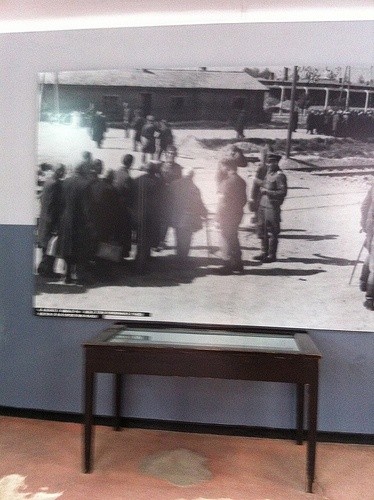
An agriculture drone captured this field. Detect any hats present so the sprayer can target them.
[264,154,282,161]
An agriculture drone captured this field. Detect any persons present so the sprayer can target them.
[34,101,374,313]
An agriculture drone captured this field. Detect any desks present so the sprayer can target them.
[79,322,323,493]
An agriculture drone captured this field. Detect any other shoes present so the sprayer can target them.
[252,252,269,260]
[362,300,374,311]
[359,282,367,291]
[261,255,277,263]
